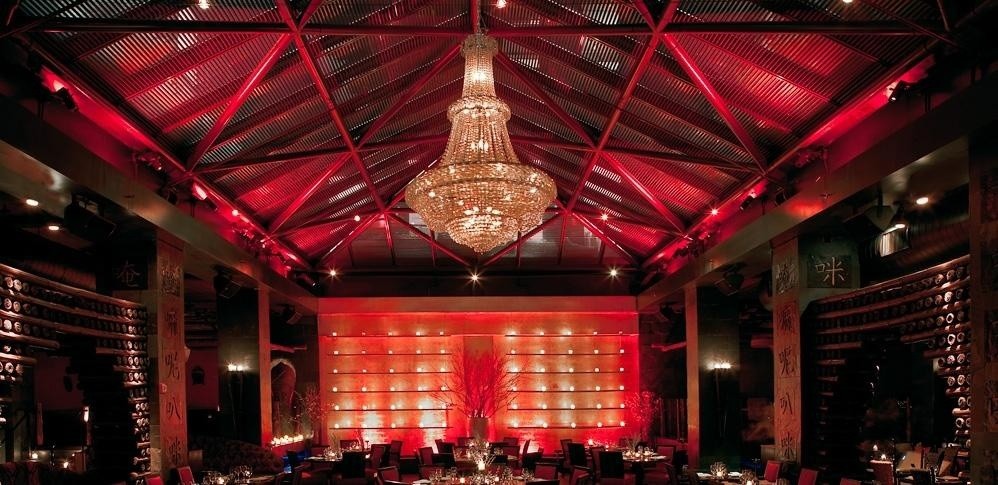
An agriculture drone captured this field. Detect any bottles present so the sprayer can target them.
[282,456,293,473]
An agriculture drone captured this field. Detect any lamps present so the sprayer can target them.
[402,0,559,261]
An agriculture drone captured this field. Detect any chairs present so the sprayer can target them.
[180,437,971,485]
